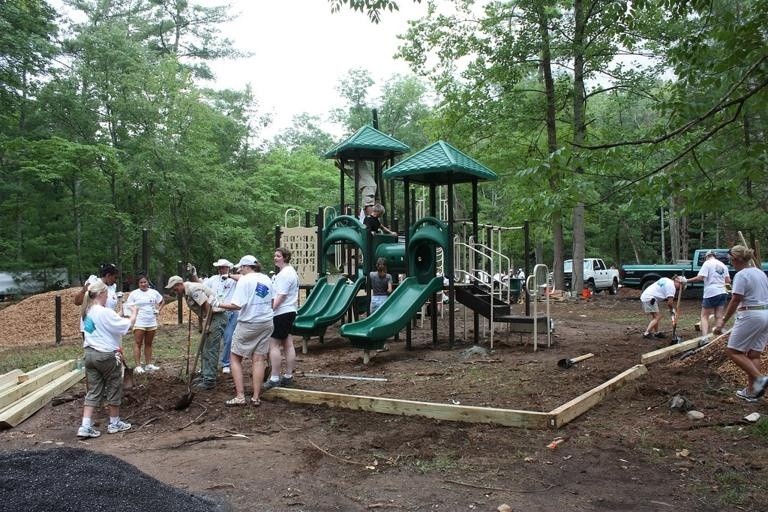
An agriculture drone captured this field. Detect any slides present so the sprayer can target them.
[289,275,365,338]
[341,275,443,349]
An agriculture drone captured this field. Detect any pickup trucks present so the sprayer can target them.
[618,248,768,294]
[547,257,621,295]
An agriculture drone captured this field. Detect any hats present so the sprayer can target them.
[269,271,275,274]
[706,250,716,256]
[165,275,183,289]
[213,255,257,272]
[89,281,107,292]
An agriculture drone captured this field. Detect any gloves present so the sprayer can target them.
[86,274,97,284]
[186,263,197,276]
[207,295,219,307]
[154,310,160,314]
[680,276,686,284]
[114,352,122,366]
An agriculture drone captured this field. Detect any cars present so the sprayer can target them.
[0,267,70,301]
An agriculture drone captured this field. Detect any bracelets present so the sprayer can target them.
[723,315,729,321]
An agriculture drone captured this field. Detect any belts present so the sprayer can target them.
[738,306,768,310]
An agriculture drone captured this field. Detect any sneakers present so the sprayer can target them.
[262,367,293,388]
[135,366,146,373]
[224,397,245,406]
[643,332,651,337]
[145,364,159,371]
[107,421,131,434]
[377,344,388,353]
[222,366,230,374]
[736,387,758,402]
[194,376,217,389]
[251,398,261,406]
[752,374,768,397]
[700,338,711,345]
[654,332,663,337]
[77,426,101,437]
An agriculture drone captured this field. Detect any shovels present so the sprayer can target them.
[670,268,685,347]
[120,352,133,388]
[679,326,735,361]
[174,303,213,411]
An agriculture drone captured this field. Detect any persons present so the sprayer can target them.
[436,268,525,315]
[364,202,398,236]
[343,160,377,215]
[640,275,687,339]
[369,258,393,353]
[207,255,275,407]
[127,276,165,373]
[712,245,768,402]
[74,265,140,438]
[165,276,227,390]
[269,271,276,283]
[187,259,241,374]
[676,250,731,336]
[263,248,300,390]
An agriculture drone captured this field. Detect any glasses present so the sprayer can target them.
[726,248,735,258]
